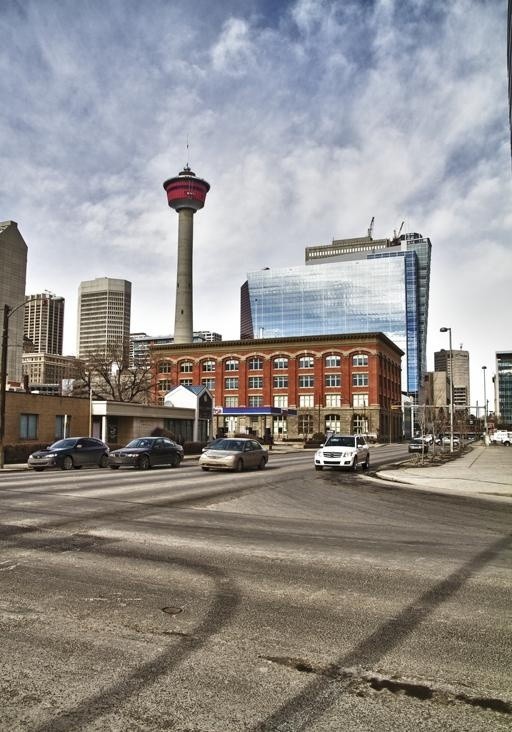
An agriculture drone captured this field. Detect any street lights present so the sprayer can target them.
[440,327,453,452]
[0,296,64,468]
[481,365,487,431]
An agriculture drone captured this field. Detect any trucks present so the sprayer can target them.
[489,430,512,446]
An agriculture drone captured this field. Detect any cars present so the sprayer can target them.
[28,437,269,471]
[408,434,459,453]
[314,434,370,471]
